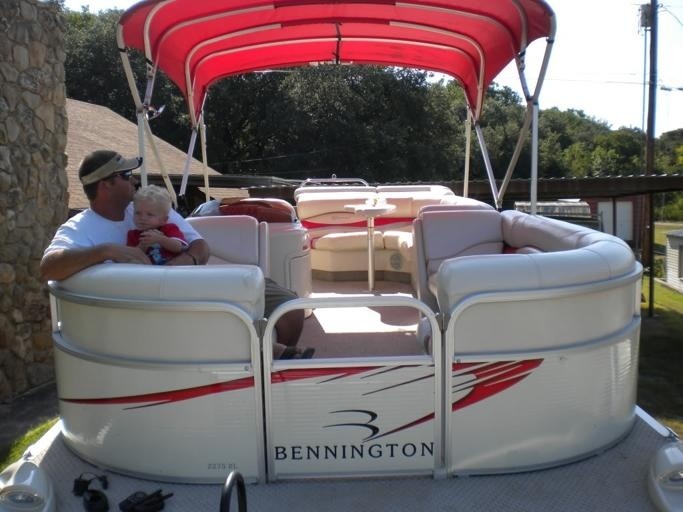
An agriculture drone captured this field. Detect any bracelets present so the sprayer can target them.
[184,251,196,264]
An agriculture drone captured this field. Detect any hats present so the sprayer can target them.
[79,150,142,186]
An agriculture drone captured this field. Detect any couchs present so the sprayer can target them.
[53,213,270,318]
[295,187,495,280]
[411,211,642,346]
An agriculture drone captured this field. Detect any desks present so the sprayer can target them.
[343,203,396,293]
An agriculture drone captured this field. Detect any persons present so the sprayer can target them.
[38,150,314,360]
[127,183,190,265]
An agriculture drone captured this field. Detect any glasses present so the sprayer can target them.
[115,170,132,179]
